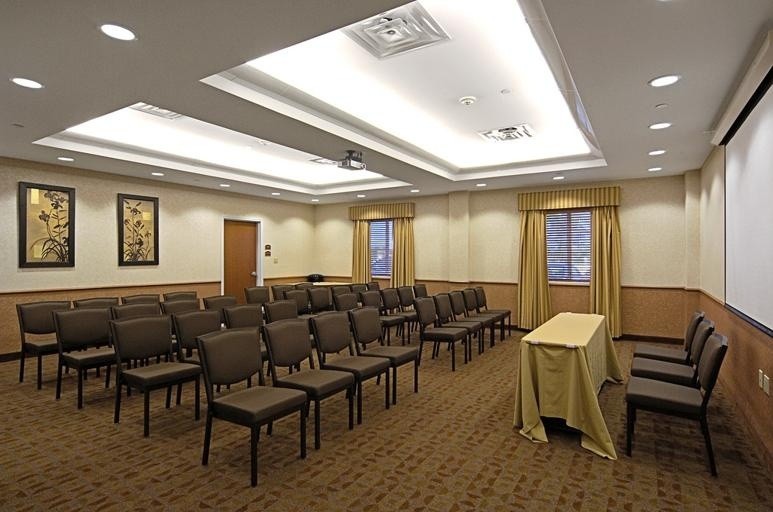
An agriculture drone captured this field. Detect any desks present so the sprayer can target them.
[520,312,607,430]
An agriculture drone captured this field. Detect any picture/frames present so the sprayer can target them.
[116,192,159,266]
[18,181,75,269]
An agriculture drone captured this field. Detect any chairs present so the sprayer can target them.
[306,286,337,315]
[15,300,87,390]
[358,290,404,346]
[72,297,119,310]
[270,284,296,301]
[432,292,482,362]
[171,308,251,406]
[333,293,383,332]
[243,285,270,320]
[111,302,159,322]
[366,281,379,291]
[309,310,390,424]
[348,305,418,405]
[120,294,159,305]
[220,302,292,388]
[108,312,202,437]
[448,290,493,353]
[630,318,715,388]
[461,288,503,347]
[294,283,313,290]
[260,318,357,449]
[162,291,196,302]
[378,288,422,344]
[412,284,427,298]
[50,307,131,410]
[411,295,468,372]
[330,285,352,306]
[282,288,318,319]
[624,331,728,478]
[159,298,200,335]
[474,286,511,340]
[262,298,328,369]
[632,308,705,366]
[201,295,237,324]
[349,283,366,303]
[396,286,416,318]
[195,325,311,487]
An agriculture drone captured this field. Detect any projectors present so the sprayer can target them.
[336,150,366,171]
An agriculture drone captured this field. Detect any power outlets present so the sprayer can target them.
[763,375,770,396]
